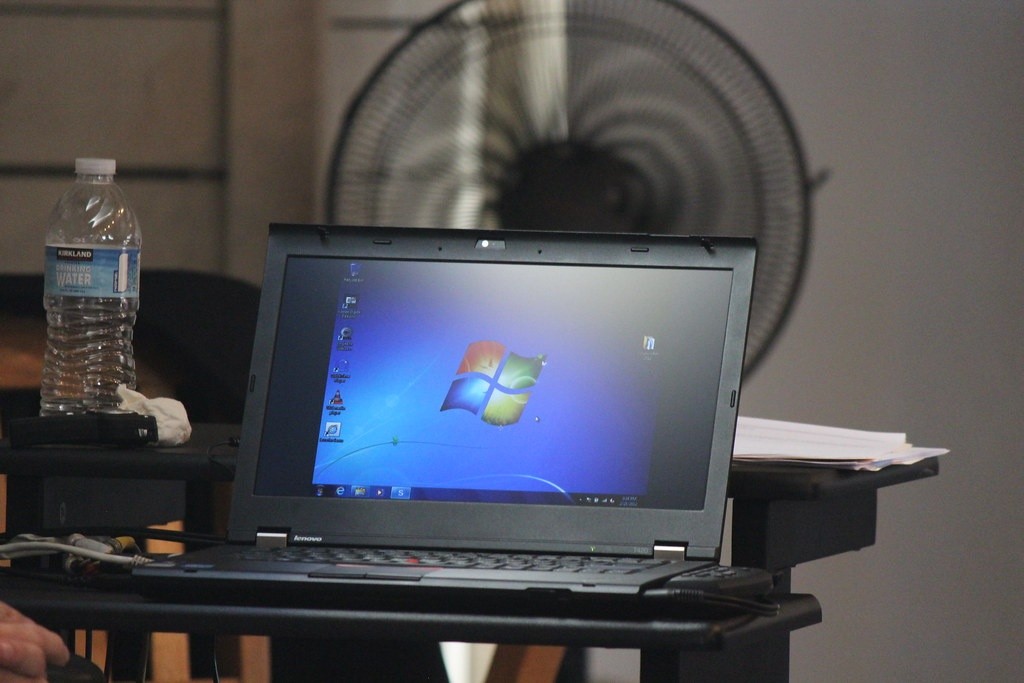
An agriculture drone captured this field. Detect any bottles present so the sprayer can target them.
[40,158,141,416]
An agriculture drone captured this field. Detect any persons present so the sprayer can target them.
[0,600,106,683]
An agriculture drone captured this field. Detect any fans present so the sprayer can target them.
[319,0,810,383]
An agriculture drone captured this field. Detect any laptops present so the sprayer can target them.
[130,221,758,613]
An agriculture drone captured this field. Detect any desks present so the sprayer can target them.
[0,422,939,683]
